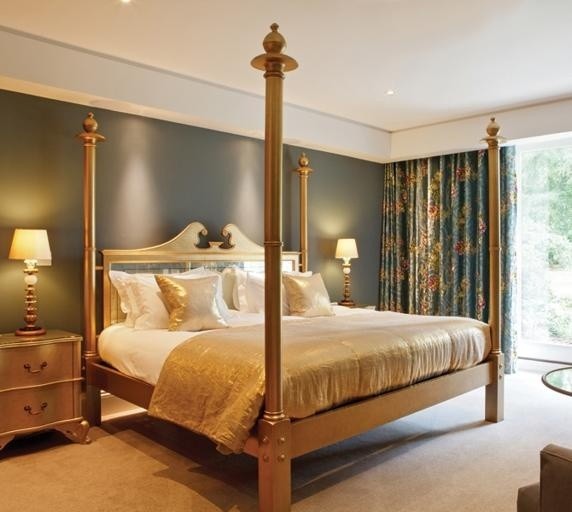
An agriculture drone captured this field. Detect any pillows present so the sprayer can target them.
[108,265,336,331]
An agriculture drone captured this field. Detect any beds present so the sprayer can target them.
[82,222,506,512]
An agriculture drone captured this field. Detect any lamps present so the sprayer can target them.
[335,238,359,307]
[8,228,53,338]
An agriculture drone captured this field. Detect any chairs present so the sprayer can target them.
[516,444,572,511]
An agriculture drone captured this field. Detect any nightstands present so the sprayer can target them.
[1,331,92,458]
